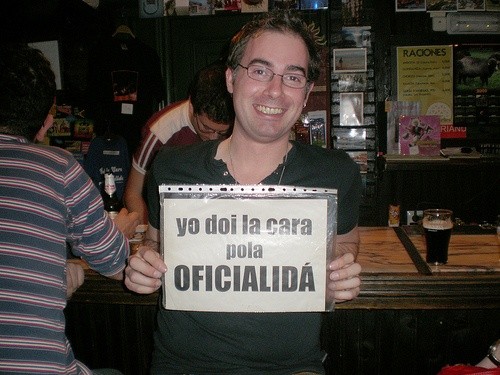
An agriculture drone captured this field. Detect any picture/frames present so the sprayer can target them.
[340,93,363,126]
[333,48,367,72]
[395,0,500,13]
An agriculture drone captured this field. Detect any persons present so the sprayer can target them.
[439,339,500,375]
[0,42,141,375]
[124,6,362,375]
[123,66,235,225]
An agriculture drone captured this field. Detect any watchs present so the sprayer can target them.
[487,344,500,367]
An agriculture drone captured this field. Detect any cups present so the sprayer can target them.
[423,209,453,265]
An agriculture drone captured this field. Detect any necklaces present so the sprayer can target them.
[228,135,288,186]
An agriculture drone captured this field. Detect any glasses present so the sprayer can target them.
[237,61,312,89]
[195,114,233,137]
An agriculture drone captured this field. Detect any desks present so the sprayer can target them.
[378,153,500,226]
[65,226,500,309]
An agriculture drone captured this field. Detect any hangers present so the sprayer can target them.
[93,9,152,53]
[91,126,126,145]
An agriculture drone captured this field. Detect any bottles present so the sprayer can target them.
[102,172,123,221]
